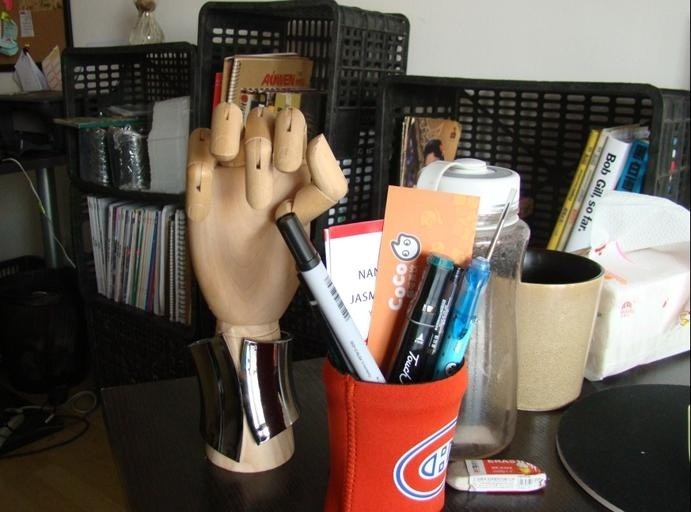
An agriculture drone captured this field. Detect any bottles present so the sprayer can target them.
[129,1,164,47]
[413,157,530,461]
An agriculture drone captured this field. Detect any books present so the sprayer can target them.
[83,192,193,326]
[320,218,387,346]
[543,121,678,263]
[211,50,327,144]
[399,115,465,187]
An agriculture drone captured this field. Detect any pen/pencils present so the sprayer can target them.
[278,212,491,384]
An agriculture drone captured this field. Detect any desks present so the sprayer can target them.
[91,317,690,510]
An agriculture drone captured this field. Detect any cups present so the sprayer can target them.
[486,250,606,412]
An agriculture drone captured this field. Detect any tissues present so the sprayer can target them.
[567,189,691,382]
